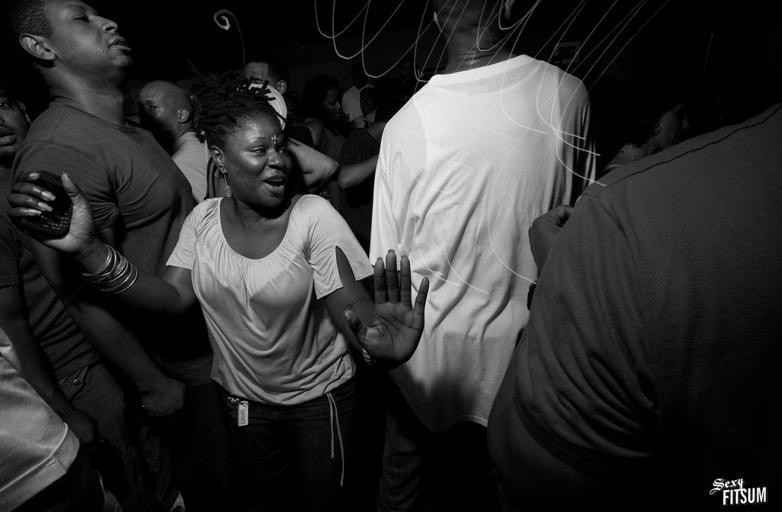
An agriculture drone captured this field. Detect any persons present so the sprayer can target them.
[1,1,781,512]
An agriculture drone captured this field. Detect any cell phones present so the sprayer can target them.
[15,170,73,238]
